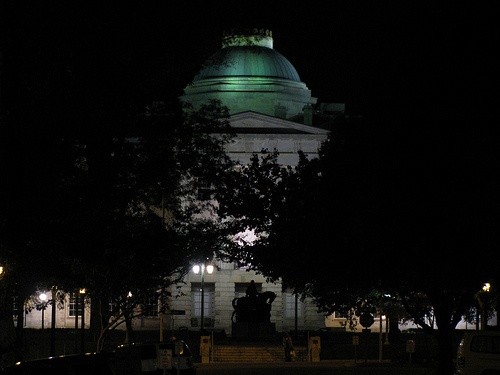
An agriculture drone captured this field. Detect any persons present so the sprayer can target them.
[237,280,271,323]
[285,335,294,362]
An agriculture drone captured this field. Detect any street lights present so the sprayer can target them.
[192,266,214,332]
